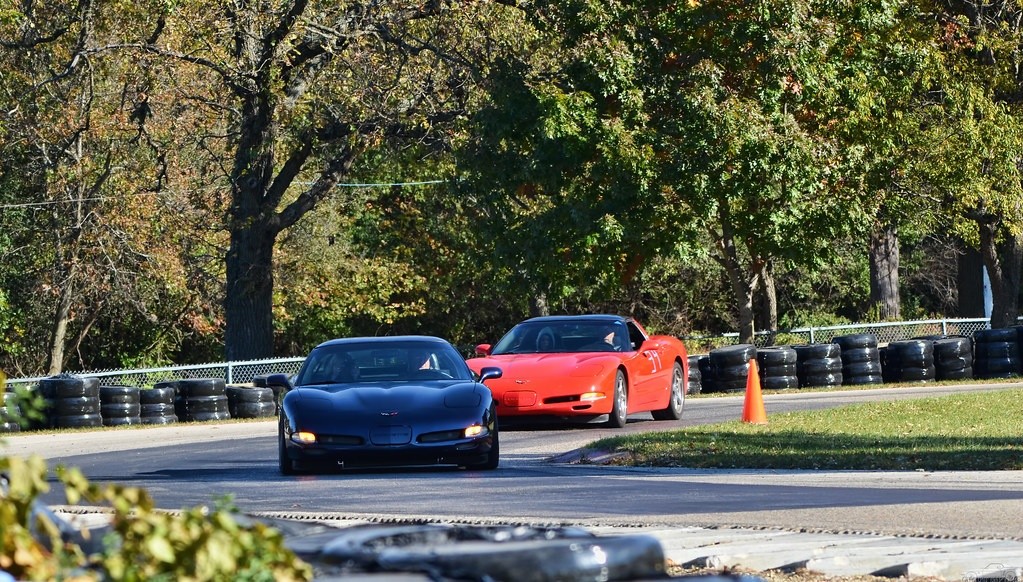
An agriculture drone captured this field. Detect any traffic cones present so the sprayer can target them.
[743,359,773,424]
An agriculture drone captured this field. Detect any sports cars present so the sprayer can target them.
[265,337,503,477]
[464,314,690,428]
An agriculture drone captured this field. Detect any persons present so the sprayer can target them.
[413,351,430,370]
[600,328,622,351]
[536,327,561,352]
